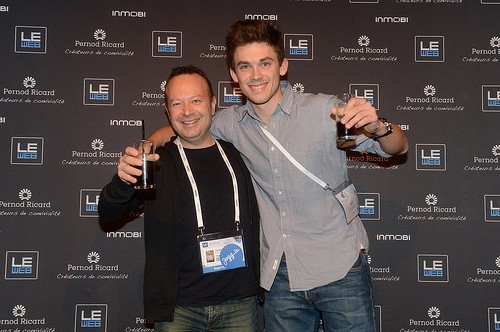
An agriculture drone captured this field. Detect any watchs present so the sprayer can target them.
[376,118,394,139]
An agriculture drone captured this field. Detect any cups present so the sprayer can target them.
[334,92,357,149]
[133,139,156,191]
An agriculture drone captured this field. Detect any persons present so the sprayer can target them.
[136,18,410,332]
[96,65,260,332]
